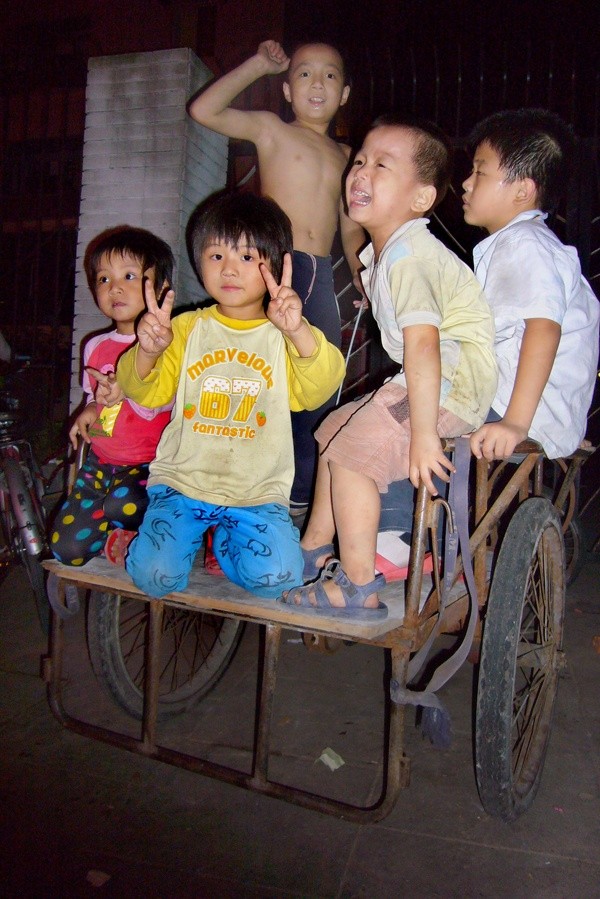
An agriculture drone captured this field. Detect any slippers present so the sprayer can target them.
[376,551,434,583]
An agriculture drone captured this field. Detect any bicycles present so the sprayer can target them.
[0,384,56,636]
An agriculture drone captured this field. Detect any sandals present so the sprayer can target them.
[299,545,386,589]
[203,525,225,577]
[276,559,389,624]
[104,528,139,571]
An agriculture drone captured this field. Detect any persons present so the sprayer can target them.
[191,39,370,534]
[48,228,175,566]
[374,113,600,581]
[105,188,346,598]
[276,114,500,618]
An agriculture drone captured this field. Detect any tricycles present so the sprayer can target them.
[38,425,598,825]
